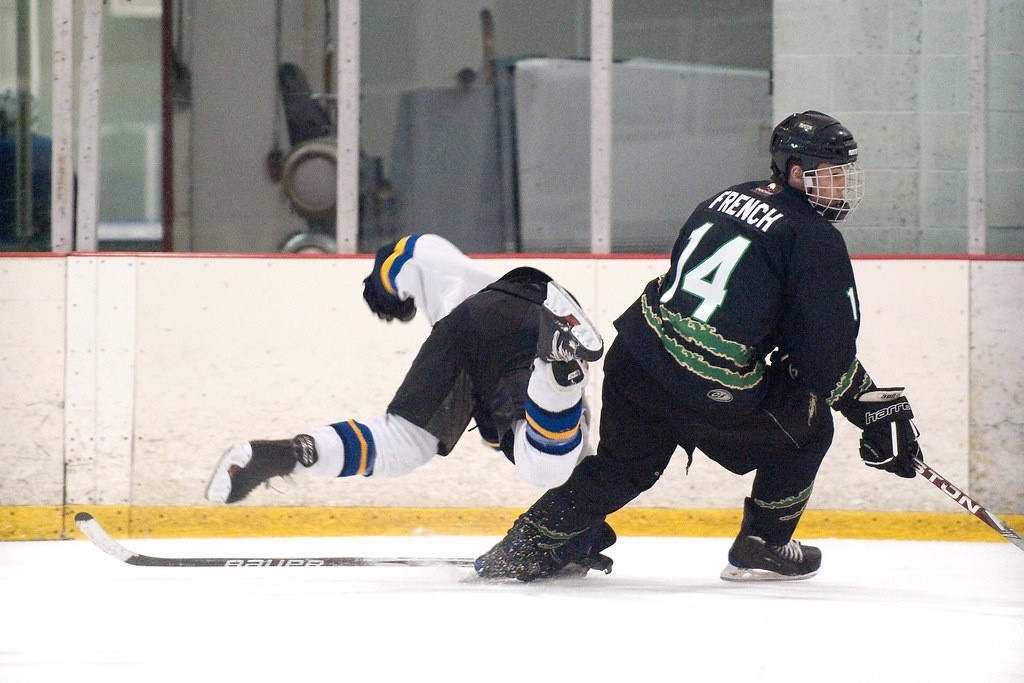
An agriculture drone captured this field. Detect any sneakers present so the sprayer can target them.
[206,434,318,506]
[721,496,821,581]
[464,516,588,583]
[534,279,604,362]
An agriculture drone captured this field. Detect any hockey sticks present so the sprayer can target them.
[908,452,1024,551]
[73,512,476,570]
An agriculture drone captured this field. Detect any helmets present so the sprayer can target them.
[770,110,864,224]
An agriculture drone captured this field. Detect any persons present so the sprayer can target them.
[473,111,924,585]
[202,233,604,504]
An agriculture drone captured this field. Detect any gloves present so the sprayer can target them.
[846,386,924,478]
[362,275,418,322]
